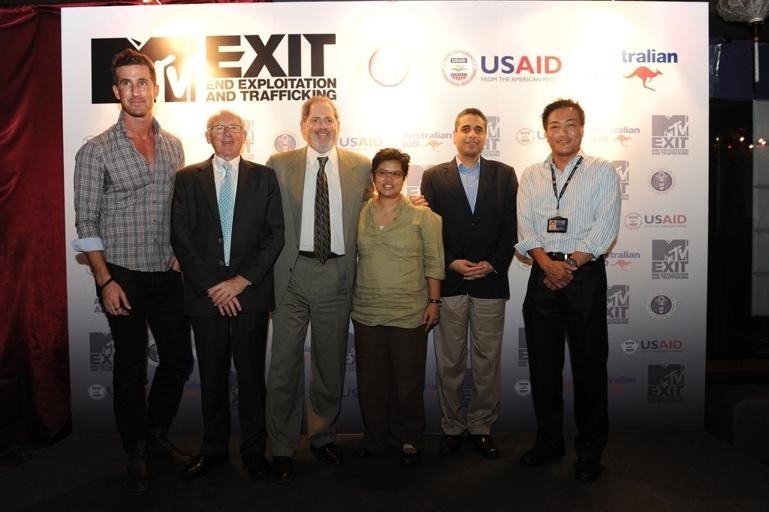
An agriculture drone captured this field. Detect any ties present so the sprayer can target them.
[218,162,235,265]
[314,157,331,265]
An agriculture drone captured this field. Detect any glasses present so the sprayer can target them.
[372,168,405,179]
[210,124,243,134]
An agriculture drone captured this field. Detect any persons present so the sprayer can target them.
[71,48,200,495]
[349,148,447,464]
[420,107,516,455]
[170,109,285,482]
[264,95,377,481]
[513,98,620,484]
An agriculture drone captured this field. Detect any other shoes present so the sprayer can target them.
[143,445,190,465]
[126,461,153,489]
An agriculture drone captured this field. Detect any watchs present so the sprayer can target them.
[428,299,442,305]
[565,257,579,270]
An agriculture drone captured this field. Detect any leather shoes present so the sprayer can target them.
[575,454,601,482]
[179,450,227,479]
[270,456,295,484]
[312,443,342,464]
[350,429,384,459]
[241,454,273,482]
[399,441,423,466]
[465,435,497,460]
[439,433,466,457]
[519,448,565,468]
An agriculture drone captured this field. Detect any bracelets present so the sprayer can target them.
[97,277,115,292]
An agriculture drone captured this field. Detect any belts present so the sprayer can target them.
[299,250,344,259]
[547,252,604,262]
